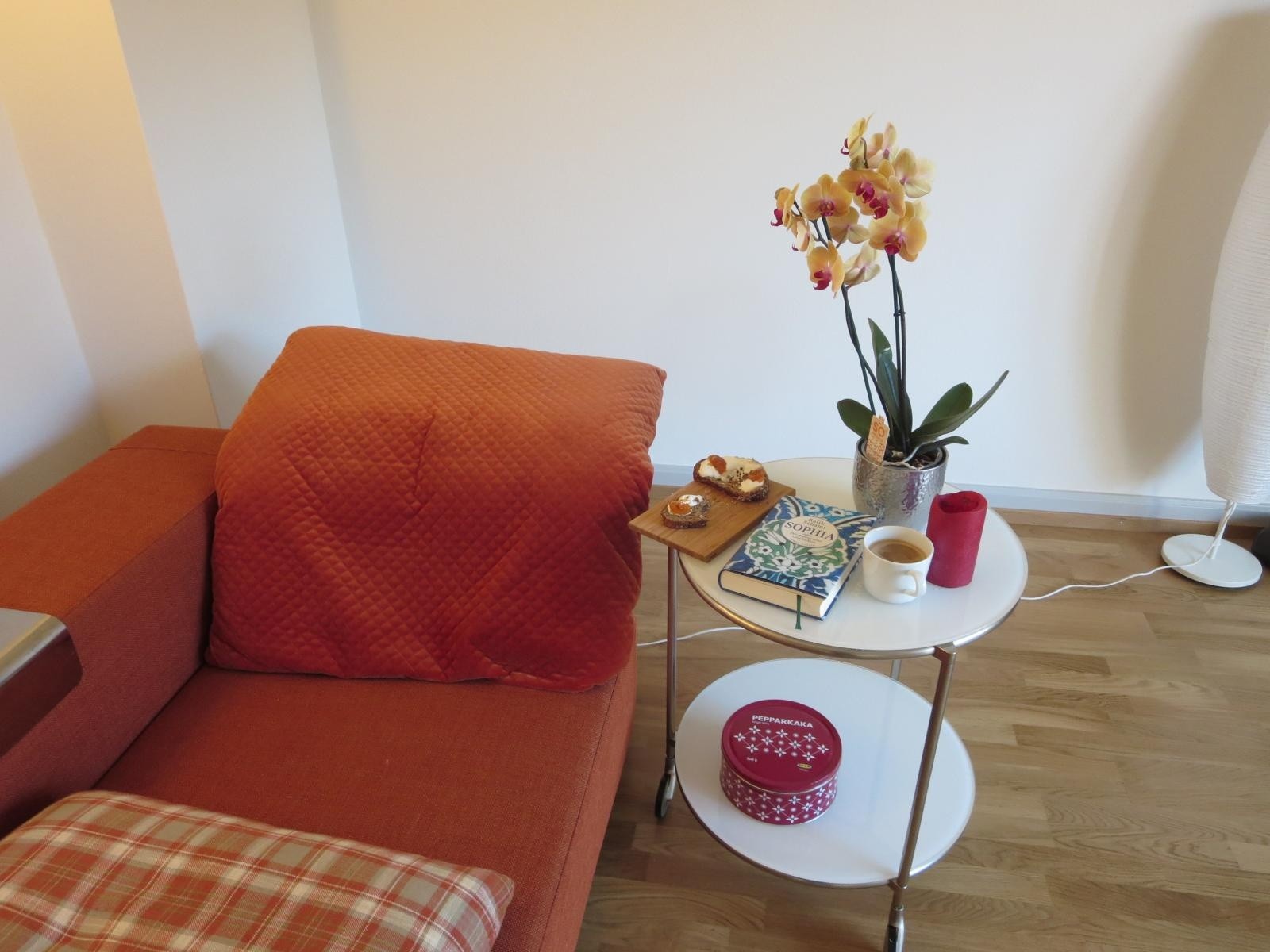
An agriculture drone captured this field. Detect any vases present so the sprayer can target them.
[852,441,951,535]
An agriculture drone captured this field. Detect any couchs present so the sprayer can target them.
[0,413,639,950]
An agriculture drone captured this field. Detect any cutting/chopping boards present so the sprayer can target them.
[627,479,797,564]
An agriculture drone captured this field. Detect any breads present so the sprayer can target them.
[694,455,768,502]
[660,495,709,528]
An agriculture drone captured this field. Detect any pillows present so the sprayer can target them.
[0,792,514,951]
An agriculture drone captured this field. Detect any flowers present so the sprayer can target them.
[768,116,1010,466]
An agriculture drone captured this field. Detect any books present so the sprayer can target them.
[718,494,877,629]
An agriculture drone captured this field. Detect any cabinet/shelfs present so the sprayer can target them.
[657,456,1027,952]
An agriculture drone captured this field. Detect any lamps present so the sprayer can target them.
[1158,132,1270,589]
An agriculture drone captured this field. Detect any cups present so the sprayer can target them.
[926,486,989,588]
[862,524,935,606]
